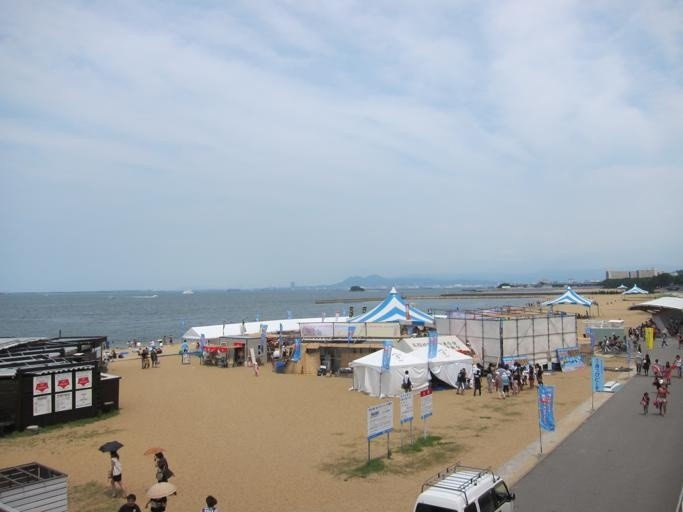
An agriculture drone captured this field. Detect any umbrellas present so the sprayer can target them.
[143,447,165,456]
[144,482,177,500]
[97,440,123,452]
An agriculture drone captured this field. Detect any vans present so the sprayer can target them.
[414,461,515,512]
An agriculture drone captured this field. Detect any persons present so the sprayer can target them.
[117,494,140,512]
[456,361,544,397]
[146,497,167,512]
[181,339,190,353]
[672,354,683,377]
[153,453,175,482]
[141,346,159,369]
[196,341,200,349]
[107,452,127,498]
[400,370,414,392]
[640,392,649,414]
[201,496,219,512]
[599,318,683,353]
[652,358,672,416]
[127,335,173,349]
[635,351,651,376]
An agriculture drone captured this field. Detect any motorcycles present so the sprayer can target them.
[656,374,665,389]
